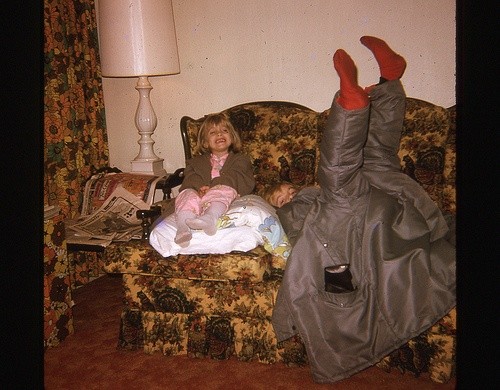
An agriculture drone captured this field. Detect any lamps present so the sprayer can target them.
[99,1,181,177]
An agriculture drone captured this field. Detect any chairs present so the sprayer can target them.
[66,168,186,289]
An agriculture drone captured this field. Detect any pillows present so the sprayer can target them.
[219,195,292,272]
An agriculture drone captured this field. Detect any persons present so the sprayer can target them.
[175,113,255,248]
[264,36,406,247]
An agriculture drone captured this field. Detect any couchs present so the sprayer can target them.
[111,99,454,383]
